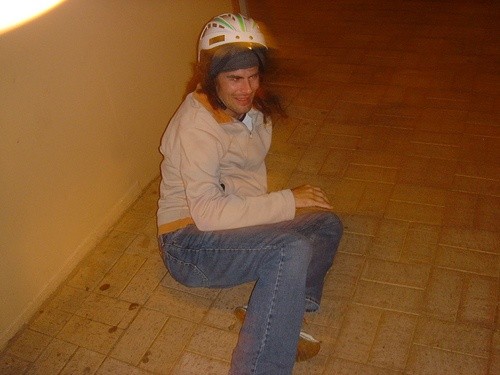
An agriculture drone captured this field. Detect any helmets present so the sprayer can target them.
[198,14,270,57]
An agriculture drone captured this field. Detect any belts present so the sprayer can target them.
[158,217,193,236]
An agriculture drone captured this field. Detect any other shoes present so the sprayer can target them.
[234,306,321,363]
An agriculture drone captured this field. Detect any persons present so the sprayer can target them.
[156,11,344,375]
